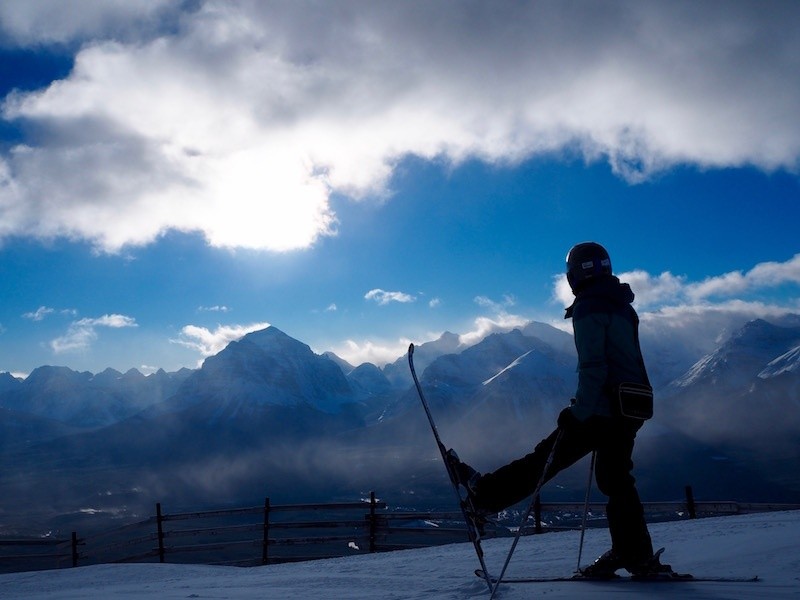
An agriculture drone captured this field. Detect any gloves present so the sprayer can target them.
[557,407,580,433]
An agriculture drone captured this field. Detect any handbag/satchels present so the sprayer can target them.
[618,382,654,420]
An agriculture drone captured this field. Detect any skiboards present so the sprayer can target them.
[408,343,761,600]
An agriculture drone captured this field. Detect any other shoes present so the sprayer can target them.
[457,462,498,517]
[583,545,657,579]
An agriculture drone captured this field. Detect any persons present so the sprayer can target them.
[446,241,653,578]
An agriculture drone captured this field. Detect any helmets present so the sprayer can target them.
[566,241,612,290]
